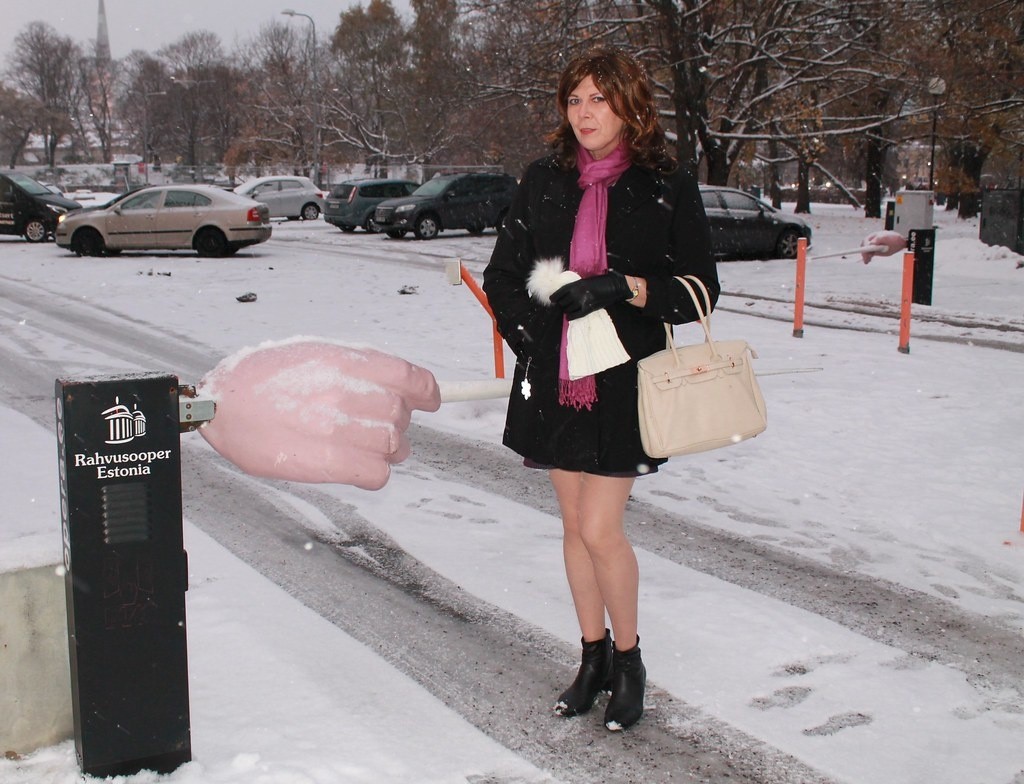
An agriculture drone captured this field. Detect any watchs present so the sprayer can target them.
[626,277,639,302]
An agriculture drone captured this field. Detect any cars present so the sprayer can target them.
[698,185,812,262]
[0,169,82,243]
[56,184,272,257]
[72,190,95,201]
[234,176,326,221]
[325,172,519,239]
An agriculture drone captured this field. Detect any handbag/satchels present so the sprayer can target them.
[636,275,768,459]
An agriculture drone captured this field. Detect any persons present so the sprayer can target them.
[482,44,721,731]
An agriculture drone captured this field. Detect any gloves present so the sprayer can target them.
[549,270,636,322]
[504,312,557,368]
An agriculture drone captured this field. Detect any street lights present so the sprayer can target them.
[282,10,317,186]
[929,78,945,191]
[128,90,167,186]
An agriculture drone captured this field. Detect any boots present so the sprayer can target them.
[555,628,613,717]
[604,634,646,731]
